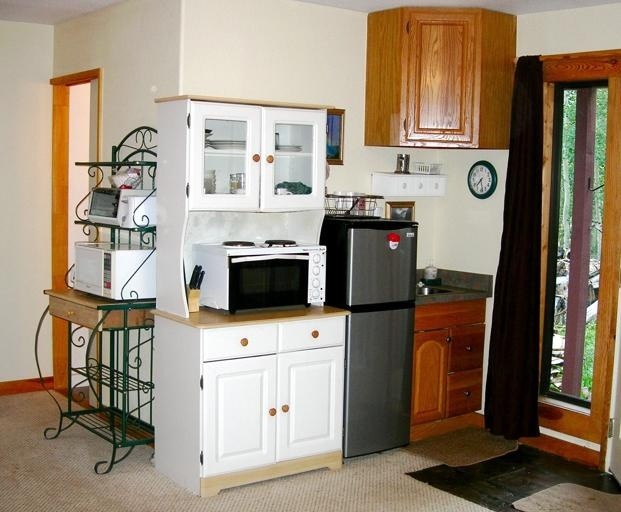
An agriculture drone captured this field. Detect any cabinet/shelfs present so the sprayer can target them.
[35,128,156,475]
[154,317,346,497]
[153,96,328,213]
[411,299,487,425]
[365,6,517,148]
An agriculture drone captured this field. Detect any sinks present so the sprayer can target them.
[417,285,467,297]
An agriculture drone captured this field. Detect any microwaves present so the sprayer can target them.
[87,187,157,228]
[73,240,157,300]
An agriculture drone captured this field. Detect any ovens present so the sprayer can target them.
[196,239,327,316]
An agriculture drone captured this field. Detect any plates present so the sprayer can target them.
[204,133,303,152]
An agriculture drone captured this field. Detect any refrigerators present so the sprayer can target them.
[319,210,419,459]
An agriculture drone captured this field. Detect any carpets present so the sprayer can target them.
[397,425,620,512]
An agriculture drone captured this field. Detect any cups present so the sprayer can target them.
[394,153,411,174]
[204,170,246,194]
[277,187,293,196]
[424,267,437,279]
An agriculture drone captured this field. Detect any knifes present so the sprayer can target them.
[189,265,205,290]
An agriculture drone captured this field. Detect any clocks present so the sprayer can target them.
[468,161,497,200]
